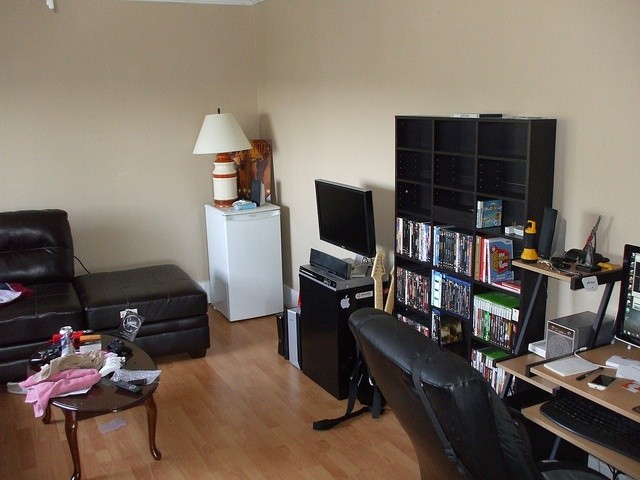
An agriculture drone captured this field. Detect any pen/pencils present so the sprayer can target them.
[576,366,604,381]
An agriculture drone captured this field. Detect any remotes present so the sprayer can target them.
[114,380,144,394]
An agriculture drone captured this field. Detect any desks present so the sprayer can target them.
[497,342,639,479]
[296,263,391,402]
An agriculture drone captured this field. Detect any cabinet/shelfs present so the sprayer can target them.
[203,201,285,325]
[310,112,558,434]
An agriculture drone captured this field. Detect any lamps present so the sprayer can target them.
[190,112,252,209]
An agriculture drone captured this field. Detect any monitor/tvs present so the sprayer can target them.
[614,244,640,349]
[315,178,376,257]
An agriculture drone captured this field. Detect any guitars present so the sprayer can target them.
[383,265,394,317]
[353,250,387,408]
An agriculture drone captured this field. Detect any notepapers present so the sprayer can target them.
[605,352,640,374]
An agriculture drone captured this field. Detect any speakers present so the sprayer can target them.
[544,311,614,360]
[537,208,557,260]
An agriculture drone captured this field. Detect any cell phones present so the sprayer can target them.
[587,373,618,390]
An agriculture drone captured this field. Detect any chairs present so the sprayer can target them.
[346,304,610,479]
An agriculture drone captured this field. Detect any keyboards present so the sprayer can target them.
[540,392,640,461]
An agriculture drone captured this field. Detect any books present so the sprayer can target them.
[434,226,474,276]
[430,270,472,318]
[472,349,517,398]
[476,200,503,228]
[396,267,431,312]
[440,319,462,344]
[473,292,521,351]
[396,313,429,338]
[451,113,502,119]
[396,217,433,264]
[474,236,514,283]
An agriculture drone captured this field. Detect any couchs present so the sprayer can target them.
[0,208,211,394]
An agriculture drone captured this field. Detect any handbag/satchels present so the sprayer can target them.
[349,343,387,418]
